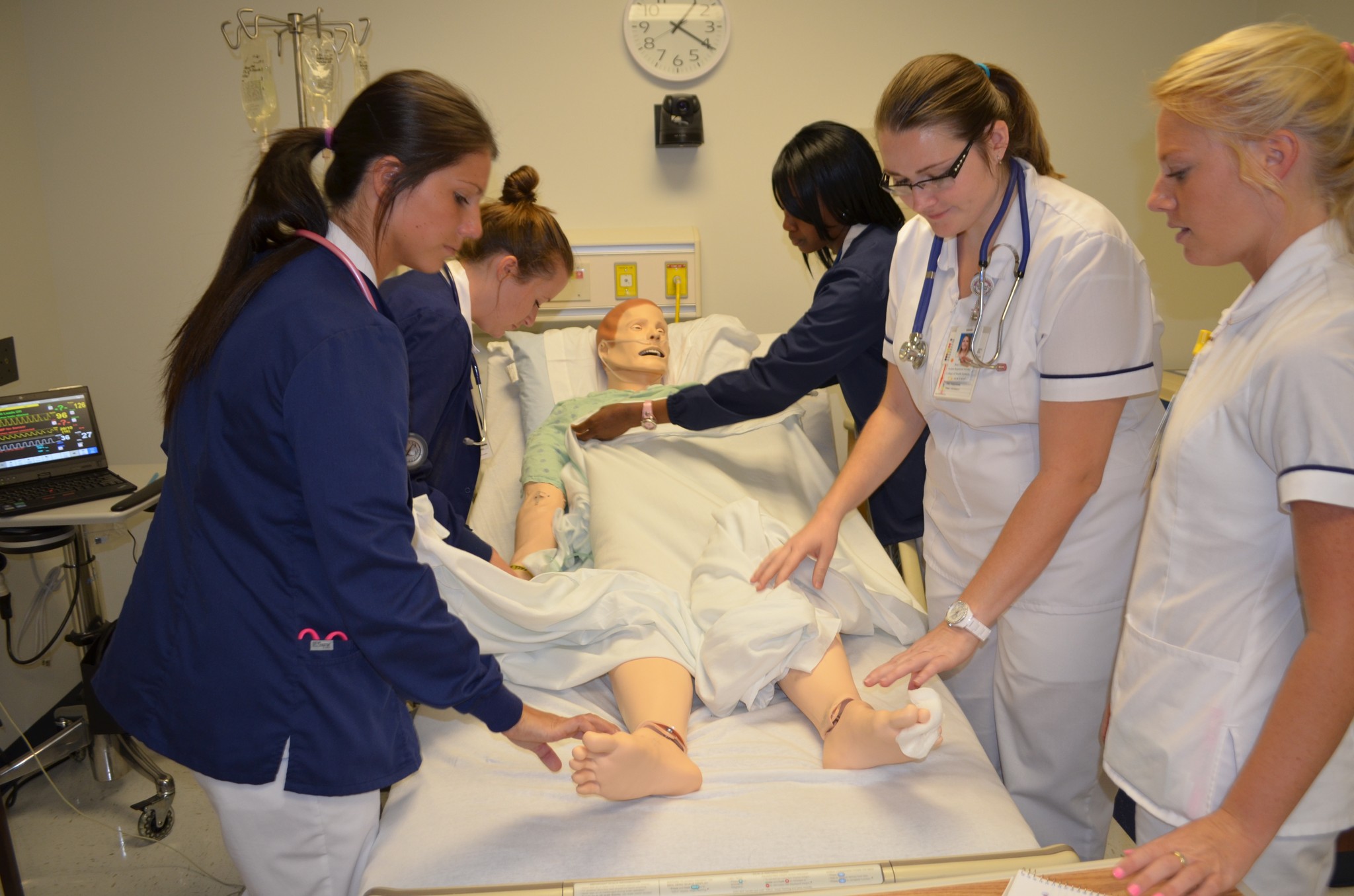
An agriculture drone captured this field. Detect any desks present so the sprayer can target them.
[0,464,176,842]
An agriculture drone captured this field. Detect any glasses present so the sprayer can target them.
[880,137,976,196]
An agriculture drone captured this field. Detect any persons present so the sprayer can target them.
[750,54,1165,860]
[955,334,973,360]
[567,121,928,547]
[509,298,944,803]
[1095,18,1354,896]
[91,67,625,896]
[377,163,576,582]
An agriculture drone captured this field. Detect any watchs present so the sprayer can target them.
[945,601,991,643]
[641,400,657,430]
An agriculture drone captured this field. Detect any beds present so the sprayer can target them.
[350,339,1084,895]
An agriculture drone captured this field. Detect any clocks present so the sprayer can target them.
[621,0,730,84]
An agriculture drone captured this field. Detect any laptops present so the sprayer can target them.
[0,386,138,516]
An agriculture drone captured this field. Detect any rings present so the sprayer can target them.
[1174,851,1186,863]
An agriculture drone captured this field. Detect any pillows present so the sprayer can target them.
[502,312,753,444]
